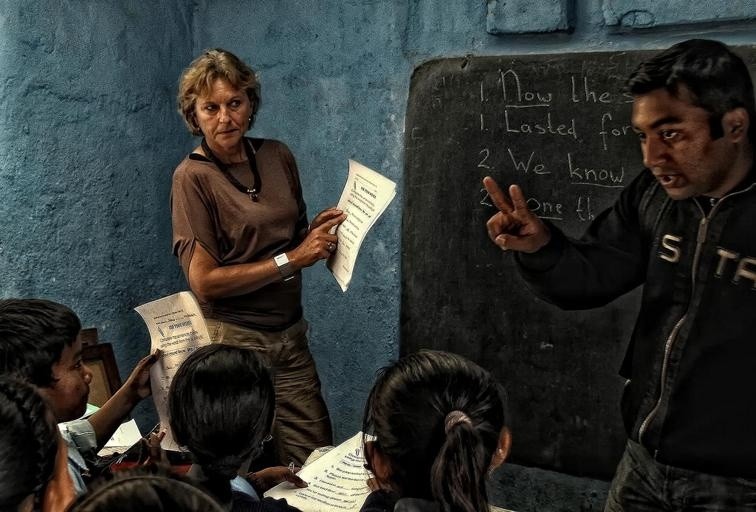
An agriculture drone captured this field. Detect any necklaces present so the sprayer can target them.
[200,138,263,204]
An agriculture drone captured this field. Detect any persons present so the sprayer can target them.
[167,342,308,510]
[360,347,515,512]
[480,37,756,511]
[0,297,164,493]
[63,464,228,511]
[168,46,348,469]
[0,371,76,512]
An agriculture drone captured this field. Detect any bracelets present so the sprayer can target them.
[273,252,295,283]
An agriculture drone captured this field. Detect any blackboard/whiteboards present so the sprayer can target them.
[400,46,754,485]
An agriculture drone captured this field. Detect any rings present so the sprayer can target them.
[327,241,336,252]
